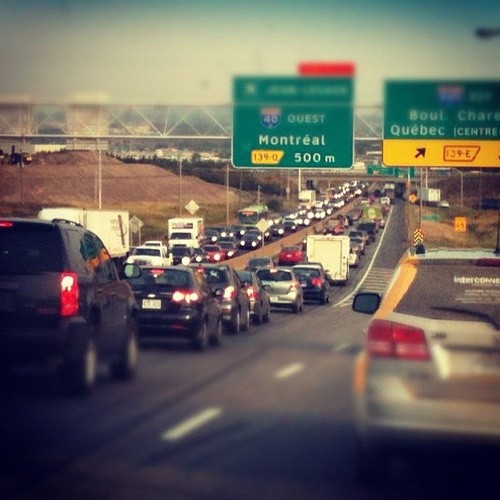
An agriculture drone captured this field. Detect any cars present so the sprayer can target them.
[255,266,303,313]
[38,180,396,271]
[128,265,224,351]
[187,263,250,333]
[235,270,272,323]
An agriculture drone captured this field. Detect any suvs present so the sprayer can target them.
[292,263,332,304]
[0,218,142,398]
[351,243,500,486]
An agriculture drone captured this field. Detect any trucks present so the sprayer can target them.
[307,235,350,286]
